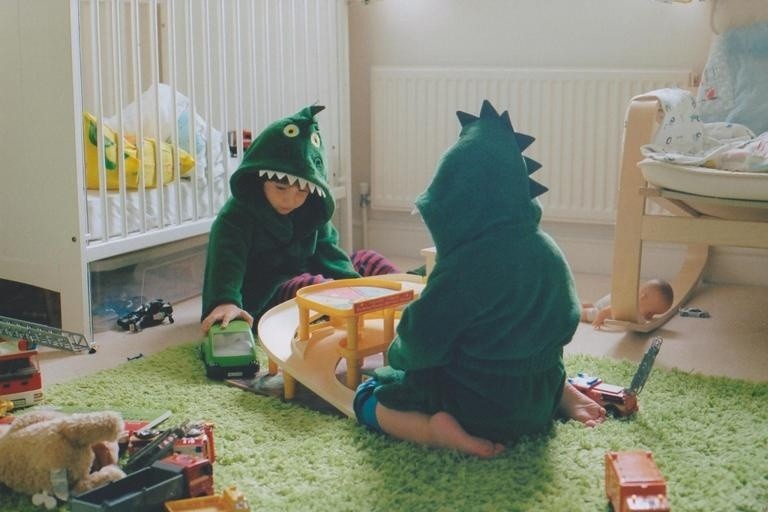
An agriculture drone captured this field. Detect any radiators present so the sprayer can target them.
[370,65,693,225]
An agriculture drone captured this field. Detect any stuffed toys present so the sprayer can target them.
[0,406,127,510]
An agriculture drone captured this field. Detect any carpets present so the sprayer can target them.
[0,336,768,511]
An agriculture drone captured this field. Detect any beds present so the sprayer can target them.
[0,0,352,343]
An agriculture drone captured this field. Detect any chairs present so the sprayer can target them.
[603,0,768,332]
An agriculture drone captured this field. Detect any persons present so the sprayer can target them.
[353,99,608,459]
[199,106,403,337]
[576,280,674,329]
